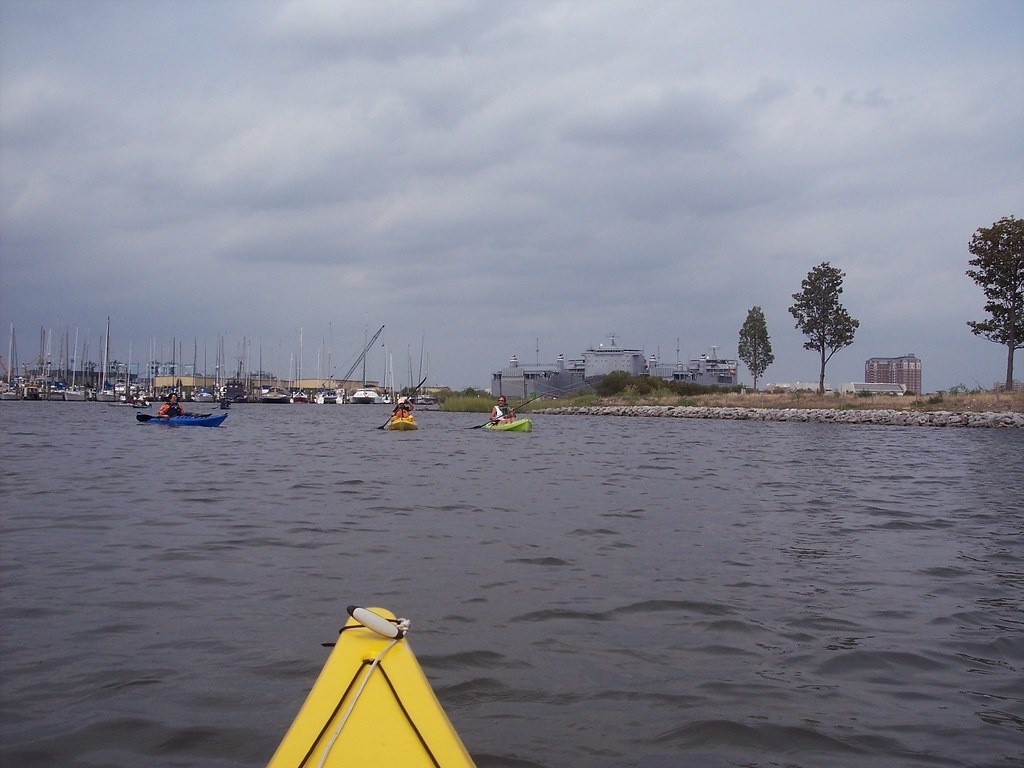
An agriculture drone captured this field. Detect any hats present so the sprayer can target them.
[398,399,405,404]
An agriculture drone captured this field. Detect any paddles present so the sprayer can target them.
[472,392,546,429]
[377,377,427,429]
[137,415,165,422]
[211,395,240,409]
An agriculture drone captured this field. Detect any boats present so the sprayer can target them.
[136,410,229,428]
[388,415,418,432]
[1,314,438,409]
[484,417,533,433]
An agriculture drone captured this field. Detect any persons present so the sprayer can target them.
[391,398,414,418]
[158,393,185,418]
[489,396,516,425]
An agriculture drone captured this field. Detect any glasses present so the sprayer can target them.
[498,399,504,402]
